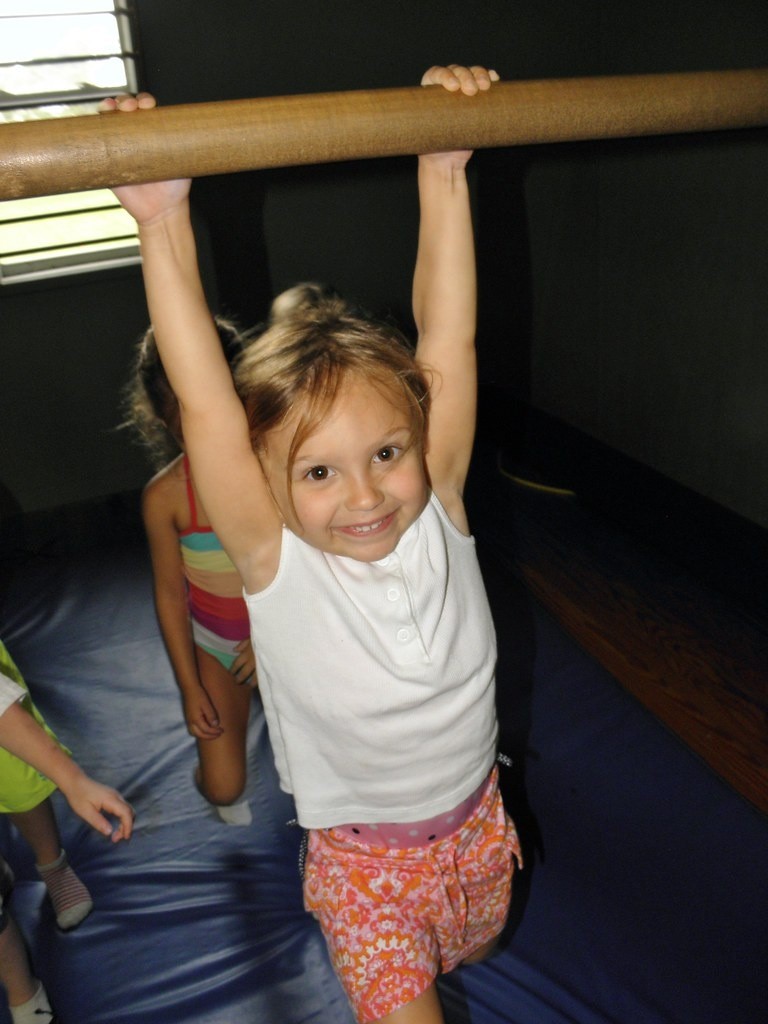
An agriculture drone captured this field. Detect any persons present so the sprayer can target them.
[0,641,139,1024]
[133,316,326,807]
[96,62,526,1024]
